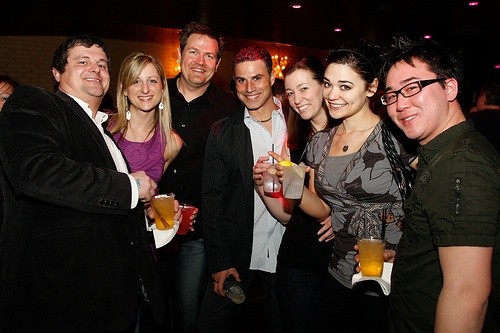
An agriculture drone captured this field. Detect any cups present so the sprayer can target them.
[150,193,175,231]
[176,205,195,235]
[263,161,281,192]
[355,236,388,278]
[283,165,308,199]
[223,275,245,304]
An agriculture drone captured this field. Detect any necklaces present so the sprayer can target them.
[337,114,375,152]
[257,105,279,122]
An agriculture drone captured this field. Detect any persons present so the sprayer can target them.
[0,35,182,333]
[353,34,500,333]
[99,23,500,333]
[0,74,19,111]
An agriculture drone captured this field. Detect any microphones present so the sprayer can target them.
[209,275,246,303]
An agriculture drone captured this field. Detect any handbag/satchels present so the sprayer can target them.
[329,279,390,333]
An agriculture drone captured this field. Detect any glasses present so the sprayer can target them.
[381,78,447,106]
